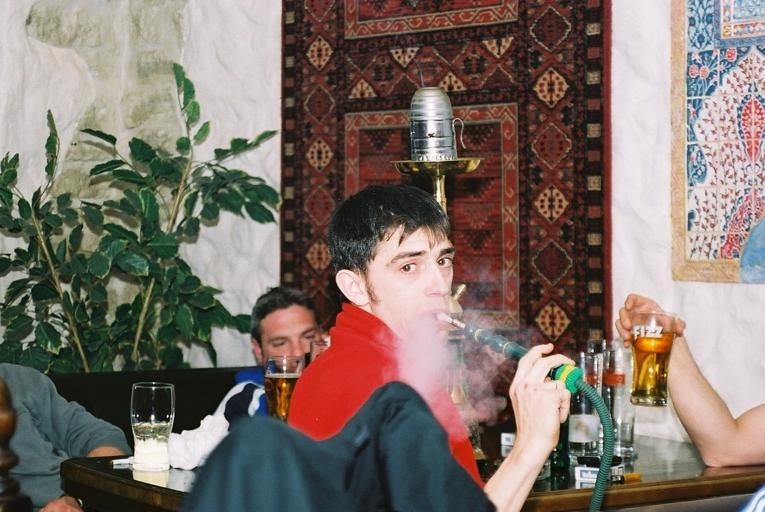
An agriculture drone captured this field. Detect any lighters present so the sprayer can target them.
[611,472,642,483]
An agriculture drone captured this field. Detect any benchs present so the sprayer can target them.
[50,367,256,454]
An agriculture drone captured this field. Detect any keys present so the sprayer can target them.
[578,455,621,468]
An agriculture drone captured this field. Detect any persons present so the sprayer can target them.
[615,293,765,467]
[213,286,329,431]
[0,362,131,512]
[287,184,571,512]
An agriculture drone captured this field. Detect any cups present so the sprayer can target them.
[265,353,304,421]
[128,380,174,471]
[564,313,675,458]
